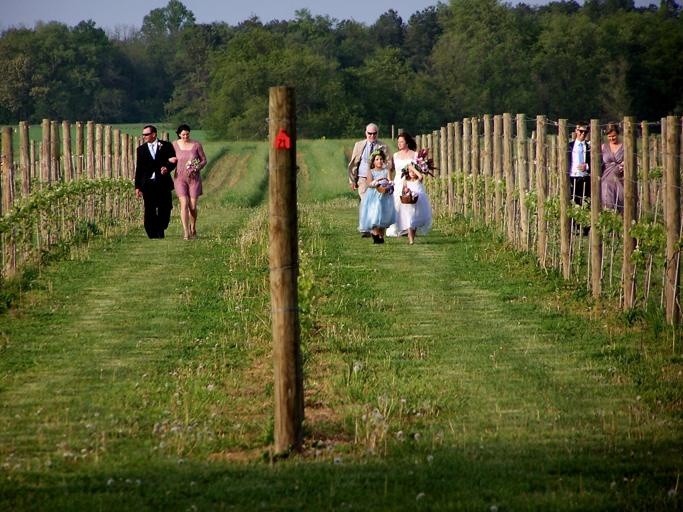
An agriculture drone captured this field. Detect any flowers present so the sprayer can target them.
[618,161,624,172]
[403,187,419,202]
[402,147,440,178]
[186,157,201,178]
[361,178,396,196]
[138,191,143,197]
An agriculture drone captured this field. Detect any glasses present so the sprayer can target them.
[367,131,377,135]
[577,129,588,134]
[142,133,153,136]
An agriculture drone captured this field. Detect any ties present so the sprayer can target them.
[151,144,156,159]
[578,143,584,164]
[370,143,373,155]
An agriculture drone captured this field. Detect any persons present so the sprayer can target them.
[356,148,398,245]
[346,123,394,239]
[396,162,431,244]
[382,132,423,238]
[166,124,207,241]
[598,127,624,212]
[133,125,178,240]
[563,121,591,235]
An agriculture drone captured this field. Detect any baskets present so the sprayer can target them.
[400,187,418,204]
[375,177,390,193]
